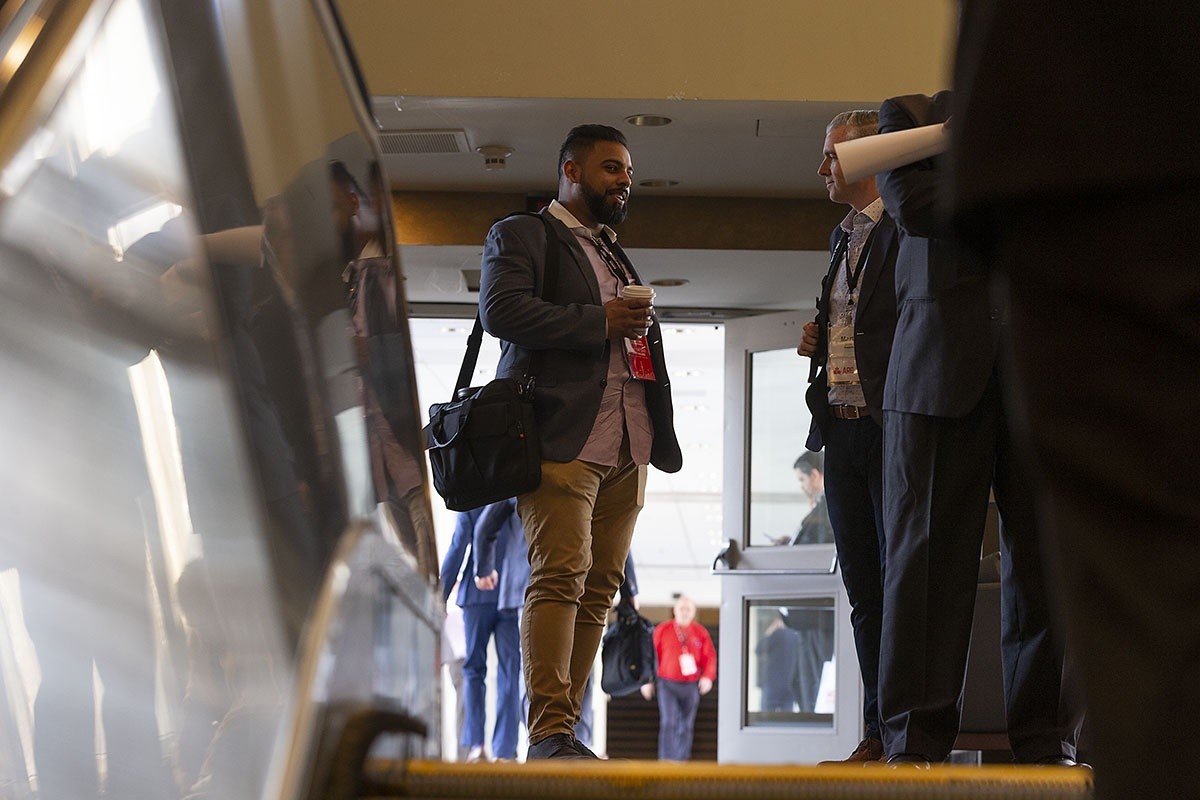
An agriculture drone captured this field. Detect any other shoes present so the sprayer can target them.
[816,738,883,766]
[465,746,487,762]
[864,753,887,766]
[494,756,517,764]
[526,734,595,759]
[1034,756,1092,768]
[887,761,921,769]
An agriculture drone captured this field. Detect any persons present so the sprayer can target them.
[796,0,1199,800]
[641,593,717,762]
[479,124,683,761]
[774,447,839,712]
[436,495,636,766]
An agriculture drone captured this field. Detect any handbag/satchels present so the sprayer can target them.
[422,377,541,512]
[601,603,656,697]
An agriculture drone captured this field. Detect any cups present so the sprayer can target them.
[622,285,657,336]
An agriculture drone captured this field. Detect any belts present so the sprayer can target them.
[830,405,870,419]
